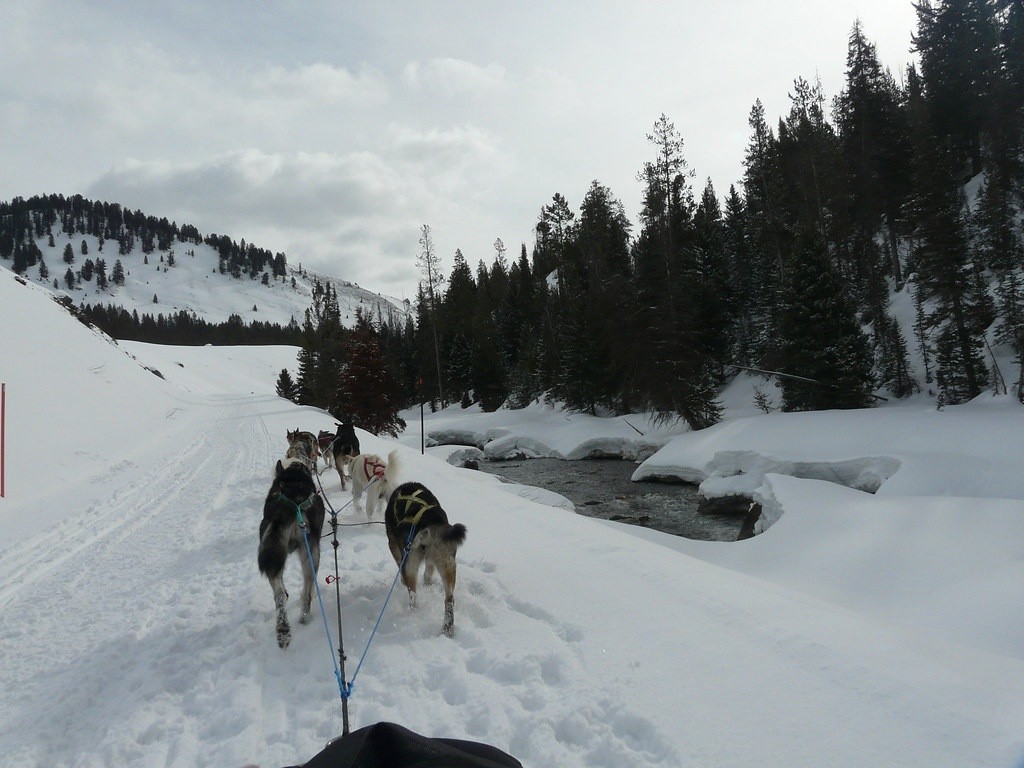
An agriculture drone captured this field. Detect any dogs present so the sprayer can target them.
[386,451,466,636]
[316,418,387,523]
[256,427,326,648]
